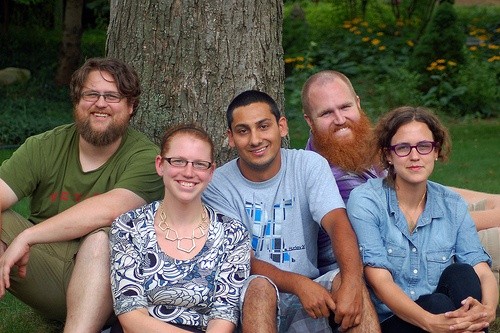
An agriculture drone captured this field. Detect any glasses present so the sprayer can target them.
[80,90,125,103]
[161,157,213,171]
[388,141,442,157]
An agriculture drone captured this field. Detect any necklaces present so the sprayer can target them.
[158,199,208,253]
[397,192,426,228]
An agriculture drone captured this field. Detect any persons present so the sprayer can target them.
[303,71,500,285]
[0,58,165,333]
[110,125,251,333]
[202,90,380,333]
[346,107,500,333]
